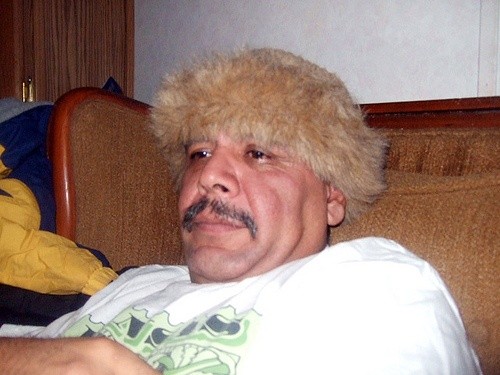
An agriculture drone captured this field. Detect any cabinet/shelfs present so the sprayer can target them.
[0,0,136,108]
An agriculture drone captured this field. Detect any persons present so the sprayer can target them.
[0,47,484,375]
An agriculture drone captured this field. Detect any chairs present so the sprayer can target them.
[49,88,500,375]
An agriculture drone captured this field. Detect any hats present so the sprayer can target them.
[147,48,391,223]
